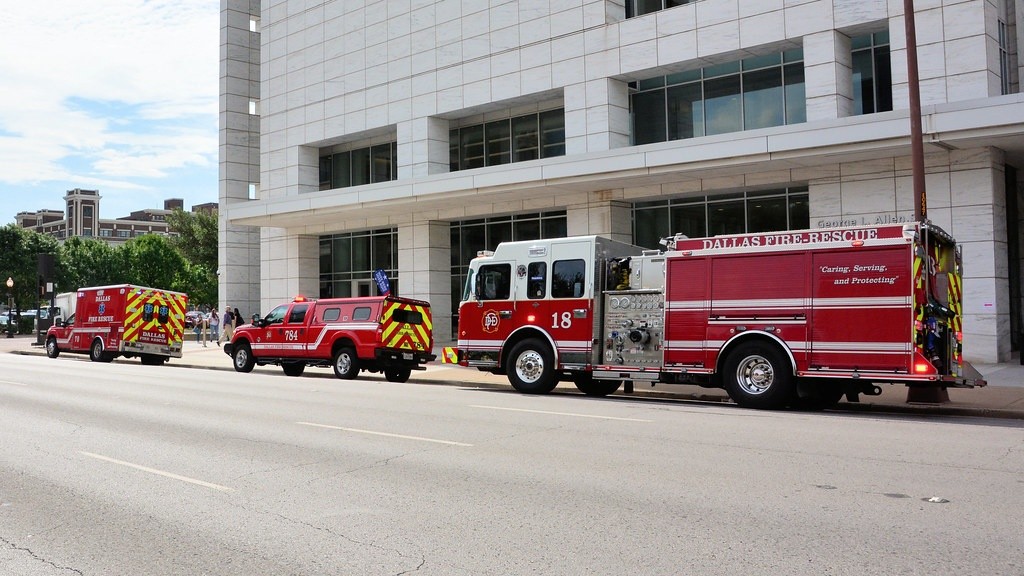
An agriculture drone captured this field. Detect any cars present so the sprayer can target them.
[203,312,220,325]
[186,310,206,326]
[0,305,39,334]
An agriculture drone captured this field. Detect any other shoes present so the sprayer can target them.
[216,342,220,347]
[197,342,202,344]
[210,340,213,342]
[217,339,218,341]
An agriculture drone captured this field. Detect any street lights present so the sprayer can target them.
[7,277,15,338]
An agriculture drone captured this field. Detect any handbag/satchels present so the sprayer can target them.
[237,317,245,326]
[193,326,200,334]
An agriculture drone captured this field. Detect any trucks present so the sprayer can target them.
[39,292,77,324]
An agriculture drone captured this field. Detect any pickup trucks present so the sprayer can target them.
[223,295,438,383]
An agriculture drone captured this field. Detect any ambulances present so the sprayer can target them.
[44,282,188,365]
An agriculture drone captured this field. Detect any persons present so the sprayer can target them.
[186,306,246,347]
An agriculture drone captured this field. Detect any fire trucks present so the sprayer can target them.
[440,220,987,407]
